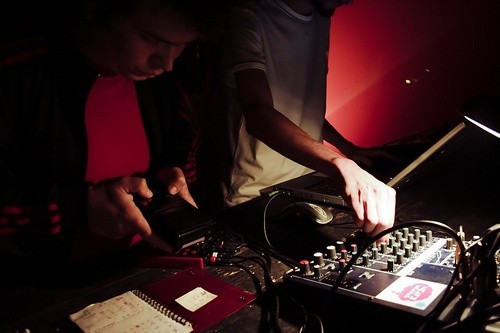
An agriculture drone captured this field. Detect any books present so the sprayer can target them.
[68,265,256,333]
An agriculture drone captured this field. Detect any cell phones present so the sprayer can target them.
[136,193,217,249]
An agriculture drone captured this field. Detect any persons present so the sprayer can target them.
[0,0,231,285]
[203,0,402,239]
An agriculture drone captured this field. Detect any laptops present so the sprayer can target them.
[273,122,467,209]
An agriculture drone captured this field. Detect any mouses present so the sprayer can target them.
[280,201,333,224]
